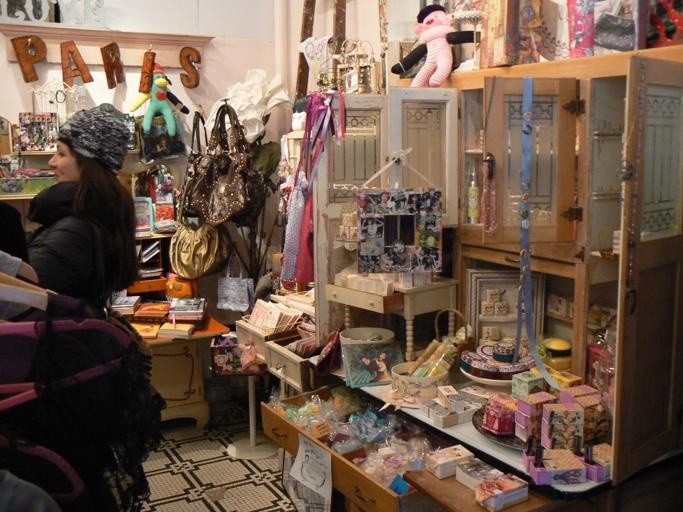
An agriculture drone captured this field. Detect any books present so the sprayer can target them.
[105,235,208,338]
[248,298,301,336]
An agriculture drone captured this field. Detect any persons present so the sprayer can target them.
[360,350,392,383]
[375,191,391,211]
[11,101,139,321]
[360,218,383,238]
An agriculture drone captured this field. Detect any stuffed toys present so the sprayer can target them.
[128,63,189,136]
[390,4,484,90]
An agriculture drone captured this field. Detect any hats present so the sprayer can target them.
[57,102,137,175]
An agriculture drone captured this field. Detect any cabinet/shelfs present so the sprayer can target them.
[235,45,682,511]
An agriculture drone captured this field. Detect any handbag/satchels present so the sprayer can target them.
[168,104,265,280]
[215,276,255,314]
